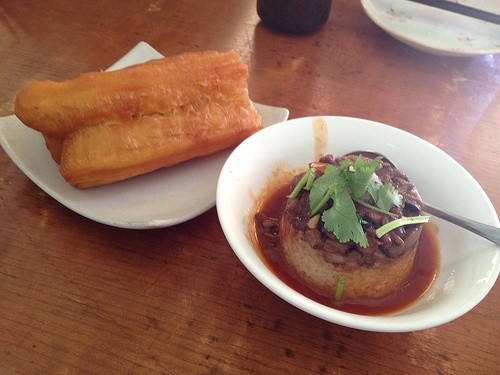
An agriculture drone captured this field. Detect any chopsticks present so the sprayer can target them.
[413,0,500,25]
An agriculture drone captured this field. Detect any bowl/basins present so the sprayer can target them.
[216,115,500,331]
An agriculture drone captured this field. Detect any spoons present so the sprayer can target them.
[342,150,500,248]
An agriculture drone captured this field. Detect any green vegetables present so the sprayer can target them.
[287,154,431,306]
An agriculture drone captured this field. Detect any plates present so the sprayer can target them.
[361,0,500,57]
[0,40,290,227]
